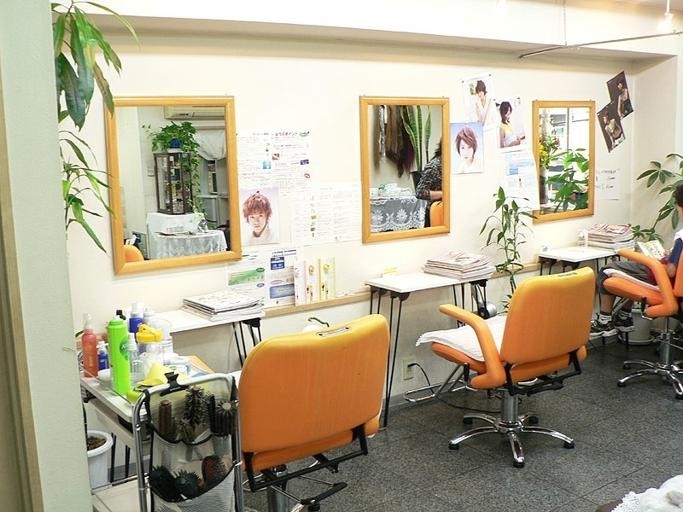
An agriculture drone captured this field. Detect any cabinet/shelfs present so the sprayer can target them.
[153,149,193,215]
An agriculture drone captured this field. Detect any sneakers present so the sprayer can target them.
[612,313,636,333]
[588,319,619,341]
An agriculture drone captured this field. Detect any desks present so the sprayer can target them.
[538,243,616,351]
[365,270,491,431]
[158,230,228,260]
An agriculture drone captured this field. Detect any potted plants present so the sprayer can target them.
[51,1,141,490]
[618,153,683,344]
[401,104,432,190]
[141,121,216,230]
[540,136,559,204]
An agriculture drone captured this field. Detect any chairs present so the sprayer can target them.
[225,314,392,512]
[603,247,683,400]
[402,267,596,469]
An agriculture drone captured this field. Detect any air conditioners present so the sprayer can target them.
[164,105,224,121]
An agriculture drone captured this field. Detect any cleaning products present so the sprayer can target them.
[81,312,99,377]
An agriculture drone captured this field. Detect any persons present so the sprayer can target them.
[472,79,498,128]
[587,184,682,338]
[453,126,478,171]
[616,81,632,120]
[415,139,444,228]
[498,101,526,150]
[602,110,624,148]
[241,192,278,247]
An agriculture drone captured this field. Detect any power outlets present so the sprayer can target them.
[402,356,416,380]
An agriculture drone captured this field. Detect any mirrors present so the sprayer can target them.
[359,95,451,244]
[103,96,241,277]
[532,100,595,225]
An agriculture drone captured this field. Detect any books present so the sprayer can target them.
[422,250,497,282]
[578,224,635,251]
[636,238,667,263]
[179,287,266,322]
